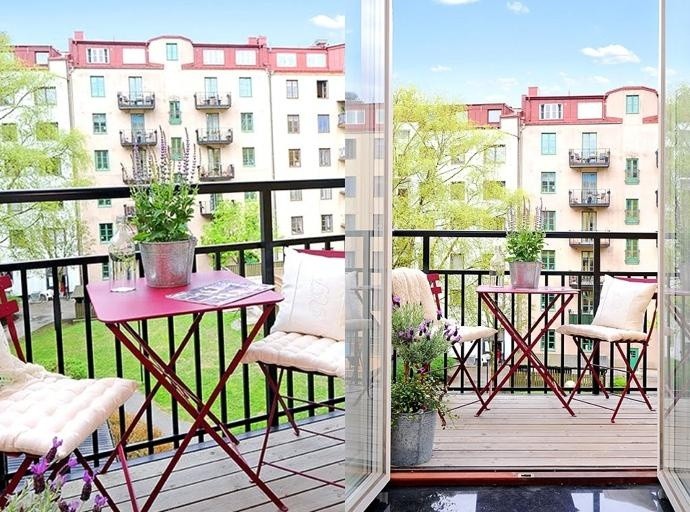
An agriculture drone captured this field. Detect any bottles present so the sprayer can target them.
[488,243,505,289]
[108,215,139,294]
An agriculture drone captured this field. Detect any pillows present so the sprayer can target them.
[590,271,658,333]
[268,247,349,343]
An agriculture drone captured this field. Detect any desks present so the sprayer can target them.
[81,267,291,512]
[471,282,585,417]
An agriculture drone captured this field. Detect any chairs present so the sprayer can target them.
[556,274,662,425]
[0,274,143,512]
[240,247,348,493]
[391,265,501,429]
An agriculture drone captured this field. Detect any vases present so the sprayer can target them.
[385,405,441,468]
[507,259,543,289]
[136,233,200,289]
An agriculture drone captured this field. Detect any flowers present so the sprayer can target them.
[0,429,112,512]
[498,194,550,263]
[385,289,464,421]
[116,120,206,243]
